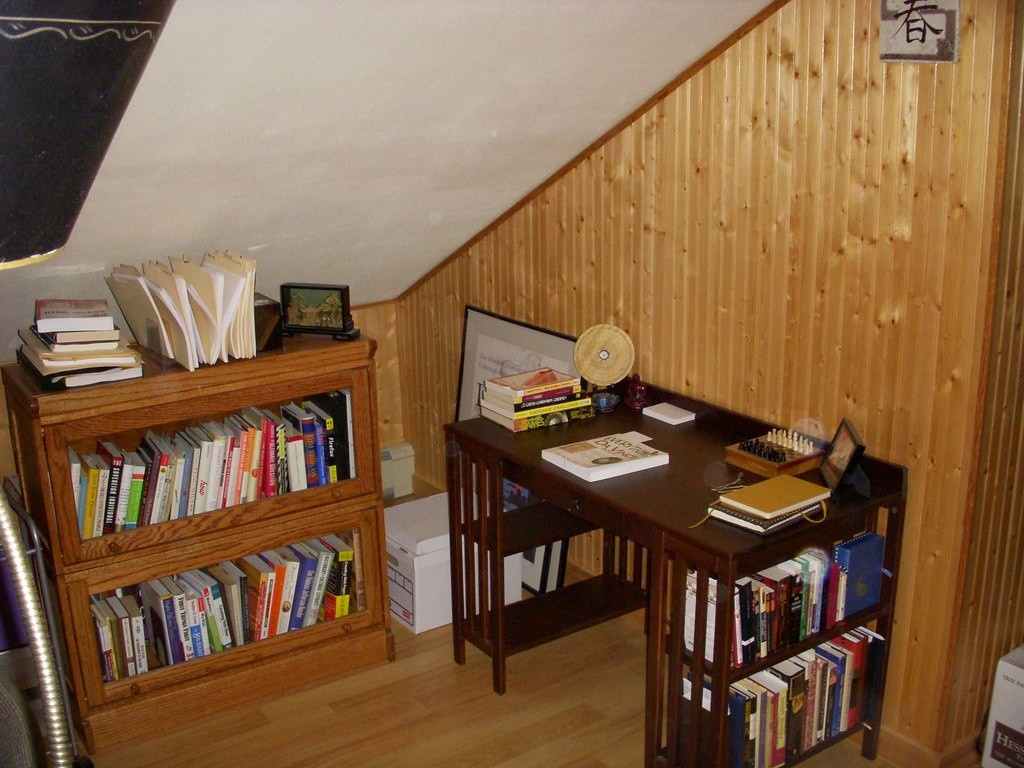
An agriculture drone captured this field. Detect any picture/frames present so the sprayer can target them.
[279,283,361,342]
[450,303,590,598]
[818,417,871,499]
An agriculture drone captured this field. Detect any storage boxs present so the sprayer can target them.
[382,487,524,635]
[982,642,1024,768]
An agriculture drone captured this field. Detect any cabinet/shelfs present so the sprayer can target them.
[0,331,396,755]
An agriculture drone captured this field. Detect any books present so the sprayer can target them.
[16,247,258,389]
[706,473,832,536]
[89,534,354,683]
[684,547,848,669]
[542,433,670,482]
[481,366,595,433]
[66,388,356,541]
[686,626,885,768]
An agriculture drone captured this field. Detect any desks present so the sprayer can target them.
[443,372,907,768]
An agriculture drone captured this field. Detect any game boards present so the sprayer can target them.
[723,433,826,480]
